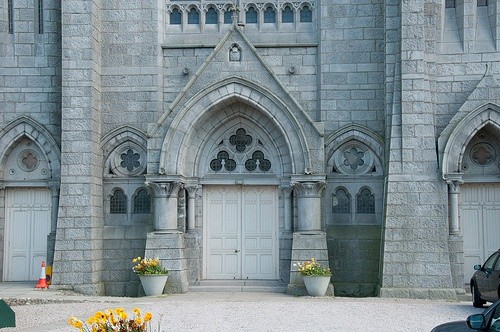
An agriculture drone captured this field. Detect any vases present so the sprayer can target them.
[302,274,332,297]
[137,273,168,296]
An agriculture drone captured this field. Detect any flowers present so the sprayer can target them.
[67,307,164,332]
[129,256,170,276]
[292,257,332,275]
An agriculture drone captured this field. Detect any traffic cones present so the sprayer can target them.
[35,261,48,289]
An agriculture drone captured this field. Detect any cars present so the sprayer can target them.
[470,249,500,307]
[429,299,500,332]
